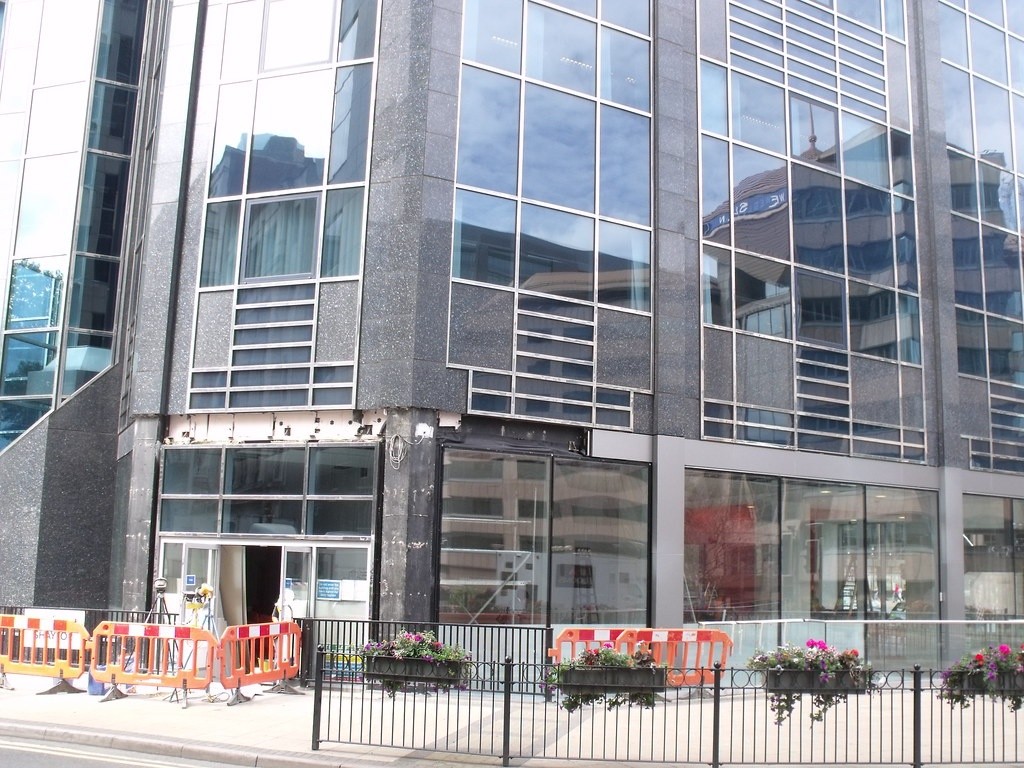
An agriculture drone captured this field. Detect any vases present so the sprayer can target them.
[563,669,666,694]
[767,669,867,695]
[964,670,1024,696]
[365,657,462,685]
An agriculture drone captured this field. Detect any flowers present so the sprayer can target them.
[747,638,880,728]
[358,630,471,698]
[538,643,669,713]
[935,643,1024,712]
